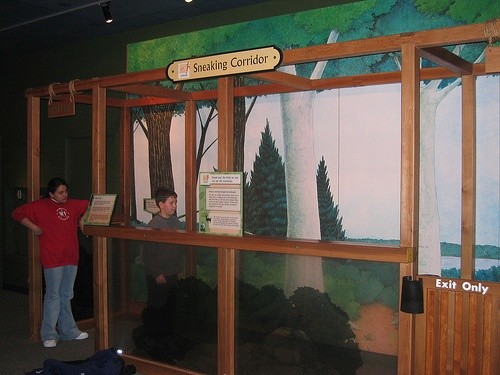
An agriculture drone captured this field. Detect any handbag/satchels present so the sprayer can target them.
[31,347,125,375]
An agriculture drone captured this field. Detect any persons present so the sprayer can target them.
[9,177,90,347]
[142,189,184,306]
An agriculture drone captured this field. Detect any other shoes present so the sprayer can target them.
[75,332,88,339]
[44,340,56,347]
